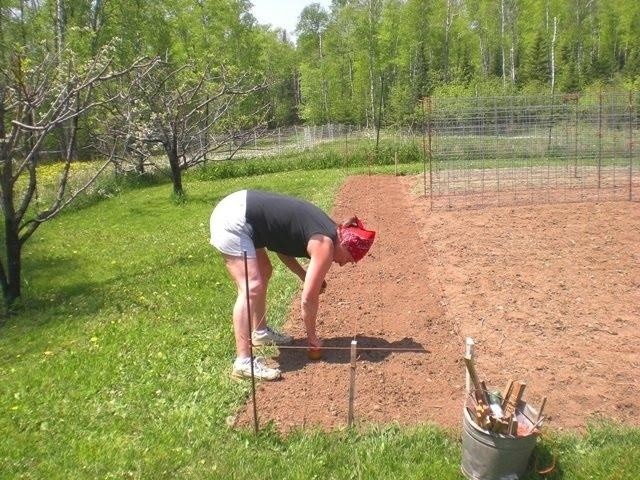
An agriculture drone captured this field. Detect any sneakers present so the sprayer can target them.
[233,360,281,381]
[252,328,294,346]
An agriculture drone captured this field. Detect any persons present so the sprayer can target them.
[210,188,376,382]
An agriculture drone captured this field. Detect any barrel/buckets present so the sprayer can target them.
[461,388,541,479]
